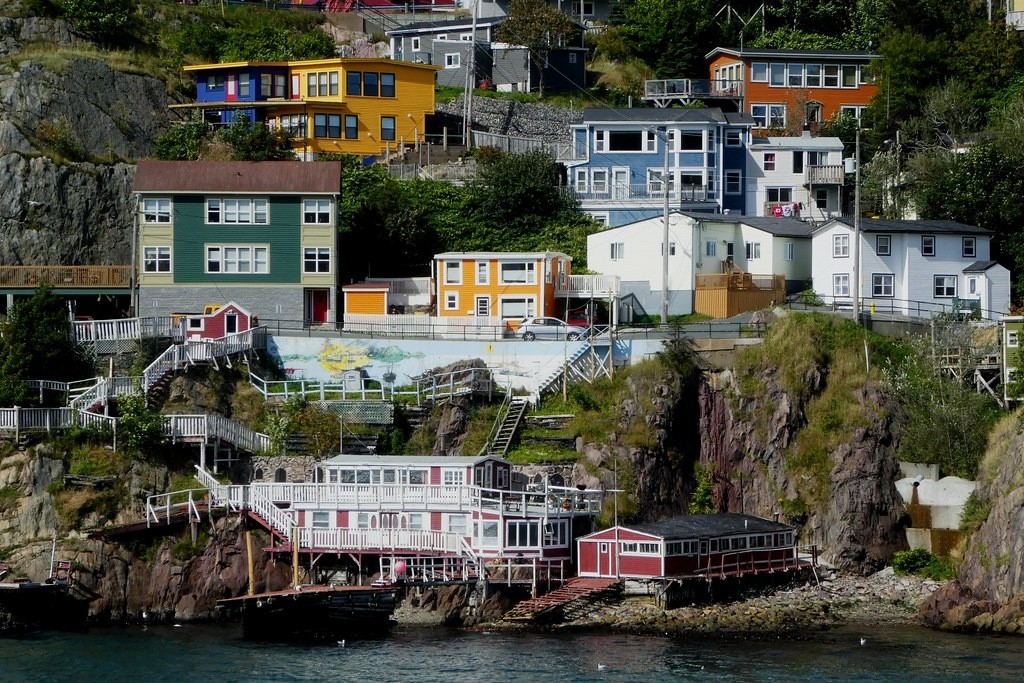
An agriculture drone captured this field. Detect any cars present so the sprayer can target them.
[567,310,596,328]
[515,317,588,341]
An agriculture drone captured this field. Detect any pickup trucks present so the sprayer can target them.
[169,305,259,329]
